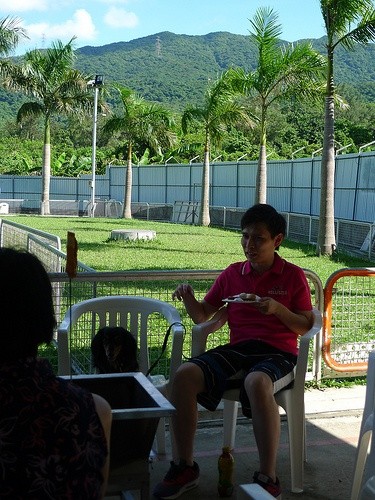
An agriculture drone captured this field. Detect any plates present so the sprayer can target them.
[222,294,261,304]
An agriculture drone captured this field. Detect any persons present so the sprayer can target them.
[0,245,112,500]
[152,204,313,500]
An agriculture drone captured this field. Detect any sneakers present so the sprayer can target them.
[249,471,282,500]
[152,460,200,500]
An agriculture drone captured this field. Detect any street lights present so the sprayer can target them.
[89,74,104,218]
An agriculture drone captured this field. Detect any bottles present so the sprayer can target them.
[218,447,235,495]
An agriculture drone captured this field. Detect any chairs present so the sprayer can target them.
[57,295,184,455]
[192,306,322,495]
